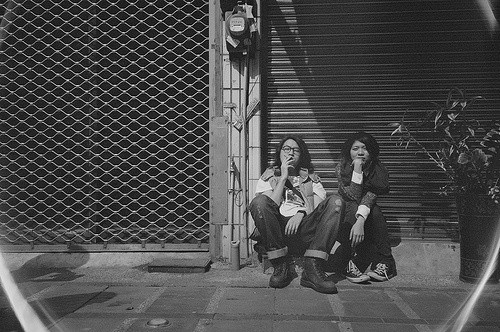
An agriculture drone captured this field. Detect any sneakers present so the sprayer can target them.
[367,263,396,281]
[340,259,370,282]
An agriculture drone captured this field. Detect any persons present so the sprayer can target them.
[248,134,345,294]
[335,133,398,283]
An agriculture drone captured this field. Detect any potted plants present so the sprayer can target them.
[386,86,500,285]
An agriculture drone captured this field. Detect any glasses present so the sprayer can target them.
[281,146,302,156]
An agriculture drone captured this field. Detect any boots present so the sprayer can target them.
[269,256,290,287]
[300,257,338,294]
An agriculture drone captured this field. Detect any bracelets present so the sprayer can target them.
[297,210,307,217]
